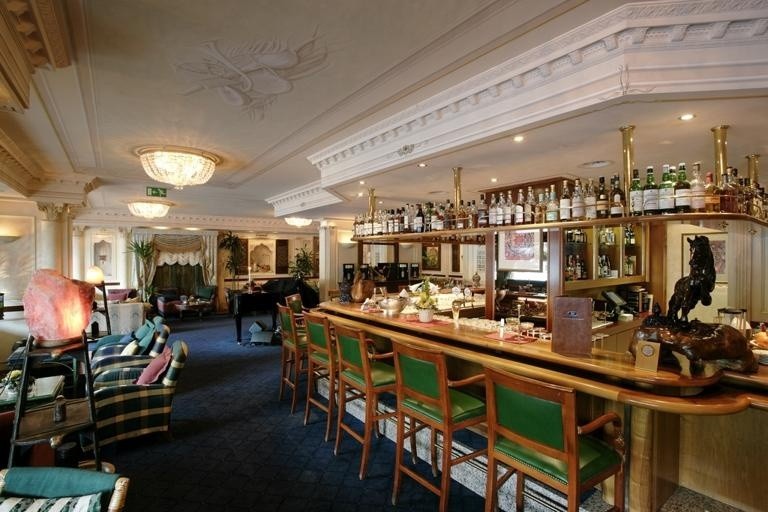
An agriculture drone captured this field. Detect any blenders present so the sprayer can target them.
[717,307,747,340]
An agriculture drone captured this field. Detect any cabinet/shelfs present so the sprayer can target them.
[563,222,653,352]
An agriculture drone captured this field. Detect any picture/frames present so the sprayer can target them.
[681,232,729,284]
[422,243,441,271]
[497,228,543,272]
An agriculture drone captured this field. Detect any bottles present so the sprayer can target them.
[353,162,768,280]
[54,394,66,423]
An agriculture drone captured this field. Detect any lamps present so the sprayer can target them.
[124,197,176,220]
[284,218,312,227]
[132,145,224,191]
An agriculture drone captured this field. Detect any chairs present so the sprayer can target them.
[154,288,214,318]
[0,315,188,512]
[108,288,136,298]
[107,303,146,335]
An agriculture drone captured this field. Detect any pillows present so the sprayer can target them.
[107,293,126,301]
